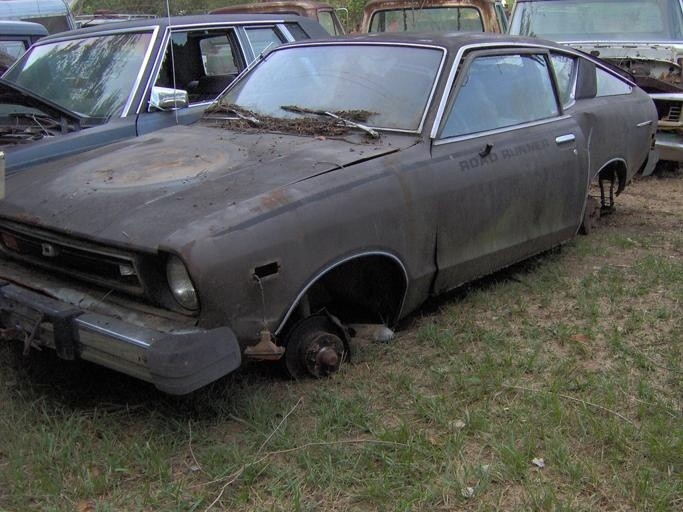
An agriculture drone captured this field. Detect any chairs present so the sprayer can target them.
[197,74,236,101]
